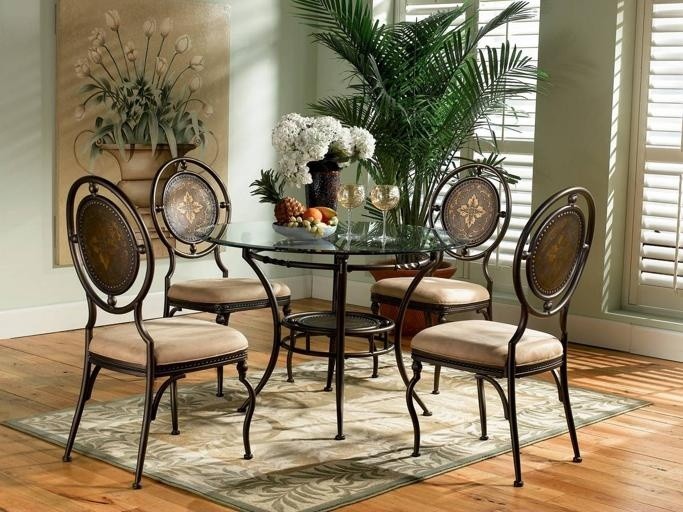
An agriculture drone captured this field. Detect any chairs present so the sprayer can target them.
[408,186,597,488]
[63,175,256,490]
[369,164,512,395]
[150,159,296,397]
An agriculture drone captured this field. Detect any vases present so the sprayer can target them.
[303,161,343,212]
[72,130,218,259]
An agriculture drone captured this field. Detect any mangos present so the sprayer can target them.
[316,207,337,223]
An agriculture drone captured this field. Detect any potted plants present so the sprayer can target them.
[290,0,552,338]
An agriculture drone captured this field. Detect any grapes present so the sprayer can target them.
[288,216,324,236]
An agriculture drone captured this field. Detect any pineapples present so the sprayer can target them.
[249,168,306,226]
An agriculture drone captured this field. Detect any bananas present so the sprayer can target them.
[328,217,338,226]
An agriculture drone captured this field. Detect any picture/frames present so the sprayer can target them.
[55,1,230,267]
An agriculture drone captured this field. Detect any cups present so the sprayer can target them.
[337,183,365,241]
[371,184,400,242]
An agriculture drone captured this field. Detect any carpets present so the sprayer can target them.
[1,348,653,512]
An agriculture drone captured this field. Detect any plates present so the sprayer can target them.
[273,221,337,240]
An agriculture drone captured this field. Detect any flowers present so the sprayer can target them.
[72,11,212,175]
[273,112,376,187]
